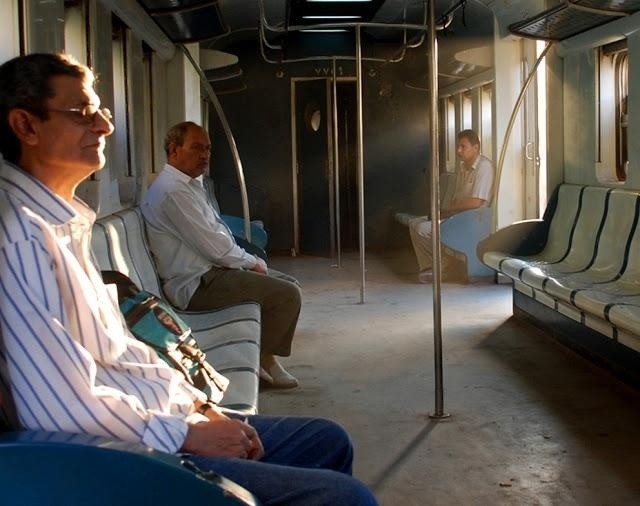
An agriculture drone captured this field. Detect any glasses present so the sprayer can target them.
[31,103,114,124]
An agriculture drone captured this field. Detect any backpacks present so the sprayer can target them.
[102,267,231,408]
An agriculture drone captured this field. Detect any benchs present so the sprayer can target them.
[475,182,640,389]
[393,171,492,285]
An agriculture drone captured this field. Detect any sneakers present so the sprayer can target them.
[260,363,299,388]
[418,270,448,284]
[395,211,427,226]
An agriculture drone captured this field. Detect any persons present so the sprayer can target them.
[139,119,303,390]
[407,130,494,285]
[0,51,381,506]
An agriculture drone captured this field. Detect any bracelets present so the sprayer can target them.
[198,403,211,415]
